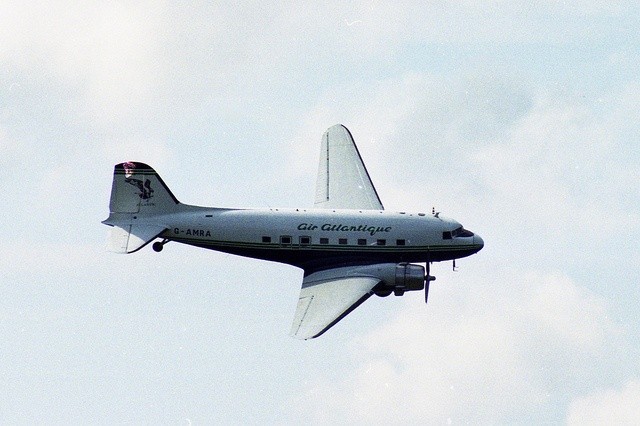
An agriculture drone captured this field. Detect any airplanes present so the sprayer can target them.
[102,124,484,340]
